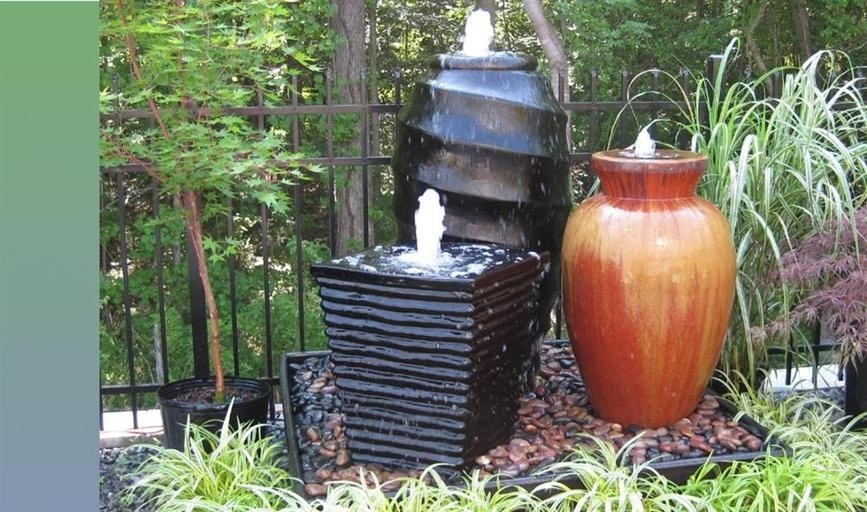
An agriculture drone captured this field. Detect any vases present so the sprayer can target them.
[393,53,572,394]
[563,150,736,432]
[310,241,545,478]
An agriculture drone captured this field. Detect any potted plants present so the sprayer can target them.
[753,210,867,433]
[100,2,327,455]
[583,35,867,401]
